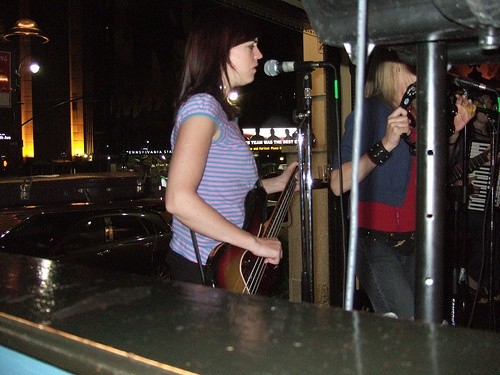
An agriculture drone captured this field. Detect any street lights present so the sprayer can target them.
[10,55,41,163]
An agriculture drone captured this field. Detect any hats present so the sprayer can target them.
[365,43,417,76]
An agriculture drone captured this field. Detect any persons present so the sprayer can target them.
[446,89,500,303]
[241,128,293,139]
[163,8,300,294]
[330,45,476,321]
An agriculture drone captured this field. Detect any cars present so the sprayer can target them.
[0,207,173,272]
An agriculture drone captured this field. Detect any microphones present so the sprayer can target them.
[445,73,496,92]
[264,60,329,76]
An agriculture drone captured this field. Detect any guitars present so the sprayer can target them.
[201,138,305,296]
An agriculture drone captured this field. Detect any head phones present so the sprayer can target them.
[399,81,458,156]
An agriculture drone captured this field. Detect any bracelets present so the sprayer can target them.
[367,140,390,165]
[449,142,456,145]
